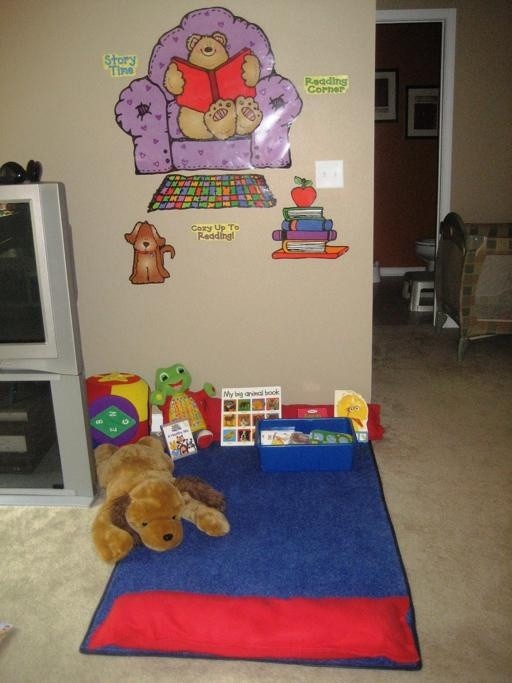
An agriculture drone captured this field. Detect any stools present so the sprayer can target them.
[403,271,436,312]
[85,371,150,446]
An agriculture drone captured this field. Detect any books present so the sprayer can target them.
[271,209,337,256]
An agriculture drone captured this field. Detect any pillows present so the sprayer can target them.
[84,591,422,663]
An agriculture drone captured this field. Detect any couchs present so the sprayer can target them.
[434,213,512,363]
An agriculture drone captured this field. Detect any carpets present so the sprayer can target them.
[76,438,423,670]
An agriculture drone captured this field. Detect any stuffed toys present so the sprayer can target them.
[91,436,229,562]
[149,365,217,450]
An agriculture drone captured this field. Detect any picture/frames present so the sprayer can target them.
[375,69,400,123]
[405,86,438,139]
